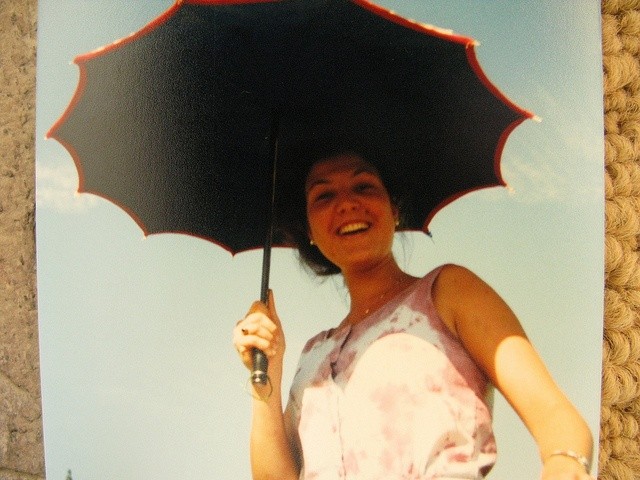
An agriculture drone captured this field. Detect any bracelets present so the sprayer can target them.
[539,450,590,474]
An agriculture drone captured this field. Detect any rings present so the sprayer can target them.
[239,325,247,333]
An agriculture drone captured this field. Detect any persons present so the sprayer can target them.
[230,141,596,480]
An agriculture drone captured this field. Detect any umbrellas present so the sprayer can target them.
[45,0,544,402]
